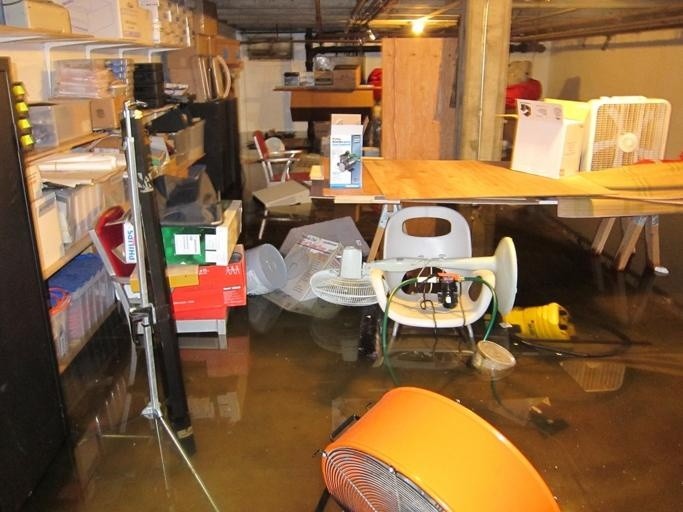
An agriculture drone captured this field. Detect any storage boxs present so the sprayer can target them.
[263,235,344,321]
[331,113,370,189]
[315,69,332,90]
[279,216,369,262]
[510,98,593,180]
[334,65,362,90]
[63,0,240,67]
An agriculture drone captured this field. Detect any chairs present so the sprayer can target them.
[253,131,311,240]
[369,206,496,355]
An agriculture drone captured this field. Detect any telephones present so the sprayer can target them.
[208,54,232,99]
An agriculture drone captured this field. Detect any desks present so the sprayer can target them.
[310,157,683,272]
[274,86,381,140]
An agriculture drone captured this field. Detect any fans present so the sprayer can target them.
[580,95,672,171]
[310,237,518,316]
[561,354,624,391]
